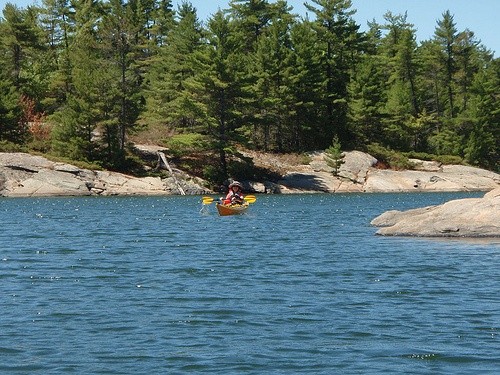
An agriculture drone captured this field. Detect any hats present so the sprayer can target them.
[229,181,243,190]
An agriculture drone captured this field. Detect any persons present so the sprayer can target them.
[219,181,243,206]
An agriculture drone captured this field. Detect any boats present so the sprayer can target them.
[216,202,249,216]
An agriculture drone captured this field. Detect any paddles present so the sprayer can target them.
[202,195,256,205]
